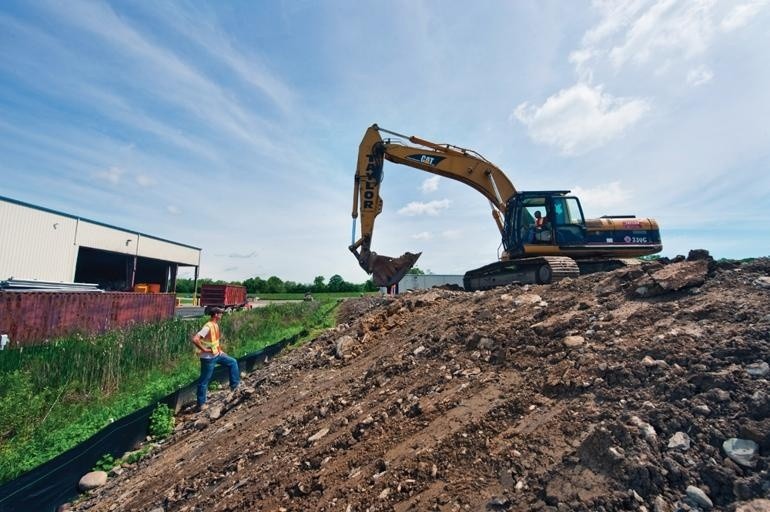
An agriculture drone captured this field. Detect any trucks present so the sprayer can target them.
[200,283,251,316]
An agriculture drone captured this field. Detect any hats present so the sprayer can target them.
[211,307,226,315]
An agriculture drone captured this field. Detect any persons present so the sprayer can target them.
[534,211,545,231]
[192,307,241,411]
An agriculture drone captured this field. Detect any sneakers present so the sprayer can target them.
[198,404,209,411]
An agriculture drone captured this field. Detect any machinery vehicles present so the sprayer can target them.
[349,123,663,288]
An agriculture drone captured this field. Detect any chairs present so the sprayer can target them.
[528,222,553,241]
